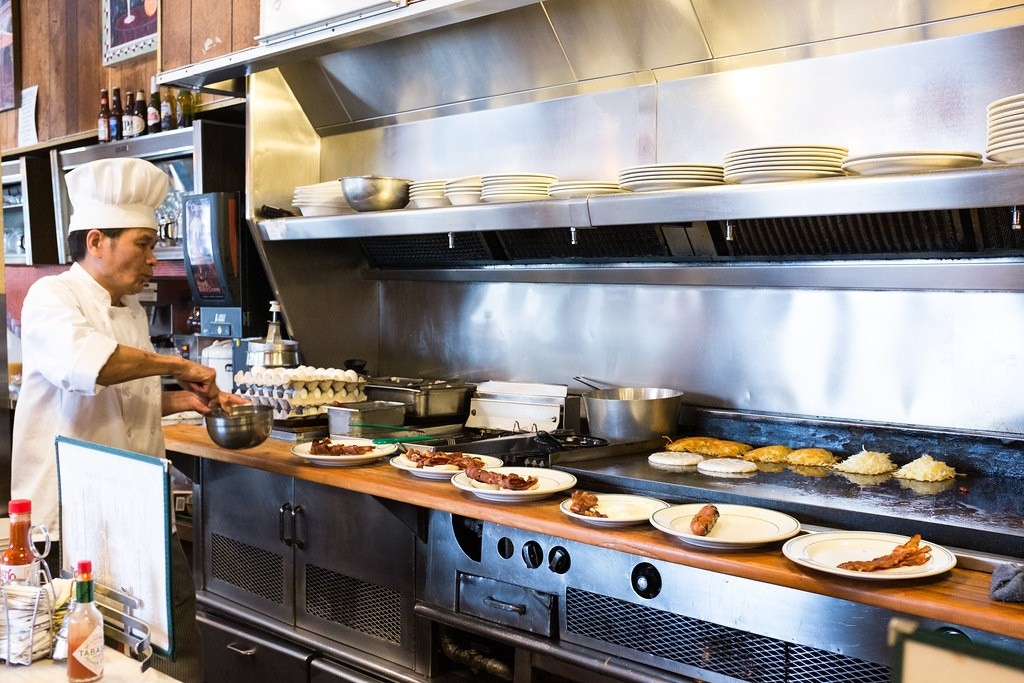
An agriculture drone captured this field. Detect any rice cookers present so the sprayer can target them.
[201,339,233,393]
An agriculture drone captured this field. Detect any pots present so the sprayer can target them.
[532,430,610,452]
[573,375,684,442]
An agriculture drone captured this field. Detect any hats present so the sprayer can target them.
[64,157,170,236]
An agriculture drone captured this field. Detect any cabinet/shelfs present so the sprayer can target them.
[0,0,263,293]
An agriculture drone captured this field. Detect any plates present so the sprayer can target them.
[450,467,578,501]
[782,531,957,579]
[390,451,503,479]
[409,154,724,209]
[290,174,359,217]
[291,440,398,467]
[559,494,670,527]
[649,503,801,549]
[724,91,1024,186]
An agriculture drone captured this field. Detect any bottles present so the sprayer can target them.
[0,499,40,587]
[53,559,104,683]
[97,75,194,144]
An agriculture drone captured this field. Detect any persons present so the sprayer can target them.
[11,157,251,683]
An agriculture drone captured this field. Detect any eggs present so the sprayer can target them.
[250,365,359,415]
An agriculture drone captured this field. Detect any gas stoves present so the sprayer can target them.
[388,380,581,454]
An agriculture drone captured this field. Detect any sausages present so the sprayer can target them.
[690,504,720,536]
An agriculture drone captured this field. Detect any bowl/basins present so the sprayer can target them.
[204,405,273,450]
[339,177,413,212]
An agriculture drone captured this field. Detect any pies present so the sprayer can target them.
[648,451,704,466]
[697,457,758,473]
